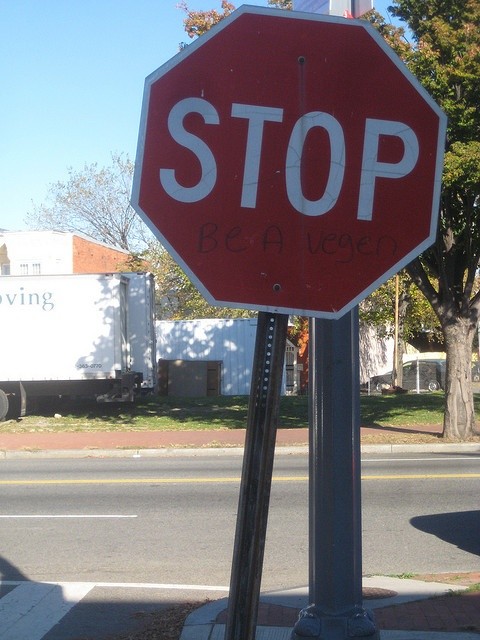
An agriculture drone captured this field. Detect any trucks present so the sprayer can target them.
[1,273,142,421]
[120,272,156,389]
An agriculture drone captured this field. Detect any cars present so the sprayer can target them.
[365,359,447,392]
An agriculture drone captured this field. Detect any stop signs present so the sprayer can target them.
[130,4,448,321]
